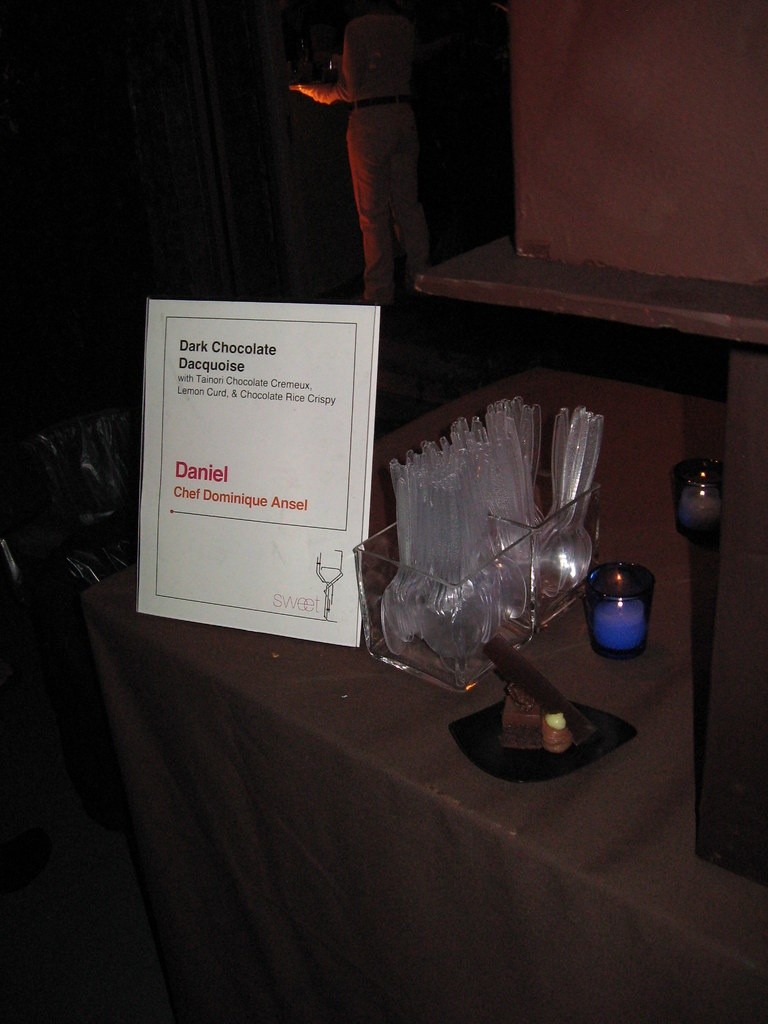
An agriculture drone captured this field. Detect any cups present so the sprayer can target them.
[672,456,723,539]
[586,562,655,662]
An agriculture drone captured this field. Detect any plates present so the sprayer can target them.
[449,697,638,784]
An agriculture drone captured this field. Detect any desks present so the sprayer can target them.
[80,368,768,1024]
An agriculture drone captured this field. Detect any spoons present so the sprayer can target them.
[381,395,604,674]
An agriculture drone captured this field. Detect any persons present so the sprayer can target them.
[288,0,430,306]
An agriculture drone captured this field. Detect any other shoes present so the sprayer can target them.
[351,295,383,305]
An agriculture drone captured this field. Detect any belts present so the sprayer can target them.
[352,94,412,110]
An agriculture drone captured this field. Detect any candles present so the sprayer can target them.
[668,456,725,541]
[585,561,655,660]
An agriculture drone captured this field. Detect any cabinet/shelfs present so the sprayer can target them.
[415,232,768,891]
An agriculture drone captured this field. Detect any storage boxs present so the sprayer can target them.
[509,0,768,287]
